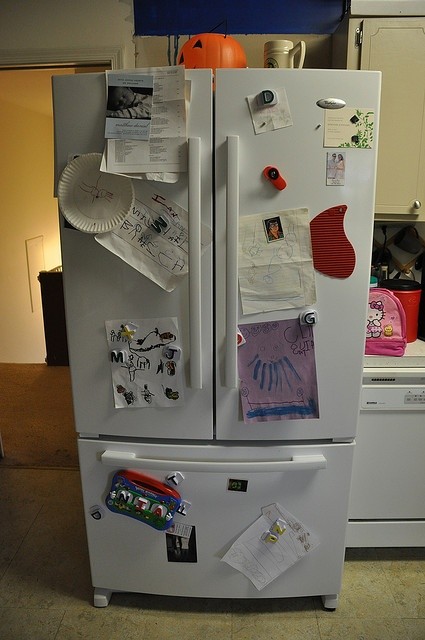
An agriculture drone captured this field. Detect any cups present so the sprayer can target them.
[264,40,306,69]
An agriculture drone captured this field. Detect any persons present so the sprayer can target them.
[106,87,153,120]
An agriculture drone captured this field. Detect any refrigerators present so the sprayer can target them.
[51,70,381,610]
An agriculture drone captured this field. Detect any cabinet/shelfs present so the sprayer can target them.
[348,17,425,223]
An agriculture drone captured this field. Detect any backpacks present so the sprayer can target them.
[367,288,407,358]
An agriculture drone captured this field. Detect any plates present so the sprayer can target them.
[58,153,134,235]
